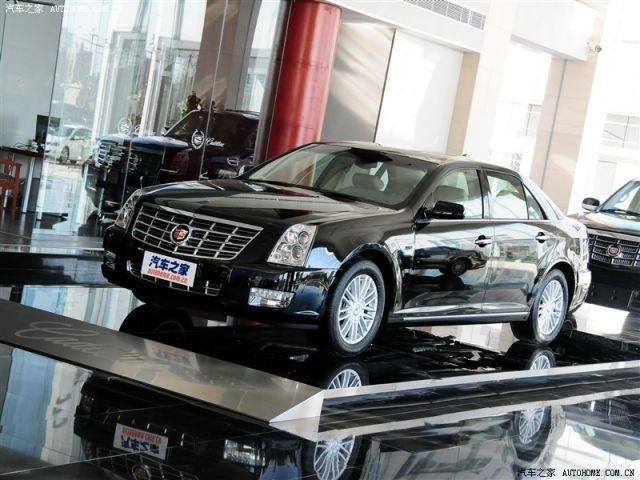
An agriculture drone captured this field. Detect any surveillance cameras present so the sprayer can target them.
[587,38,602,52]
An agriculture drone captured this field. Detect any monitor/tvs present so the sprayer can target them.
[35,115,61,152]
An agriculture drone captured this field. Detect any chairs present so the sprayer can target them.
[495,190,522,199]
[393,178,433,209]
[0,157,23,222]
[434,186,467,202]
[340,172,384,200]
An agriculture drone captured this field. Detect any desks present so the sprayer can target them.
[1,145,59,221]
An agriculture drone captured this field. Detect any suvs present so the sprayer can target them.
[84,105,260,211]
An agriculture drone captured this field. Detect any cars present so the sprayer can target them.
[73,376,565,480]
[45,124,92,165]
